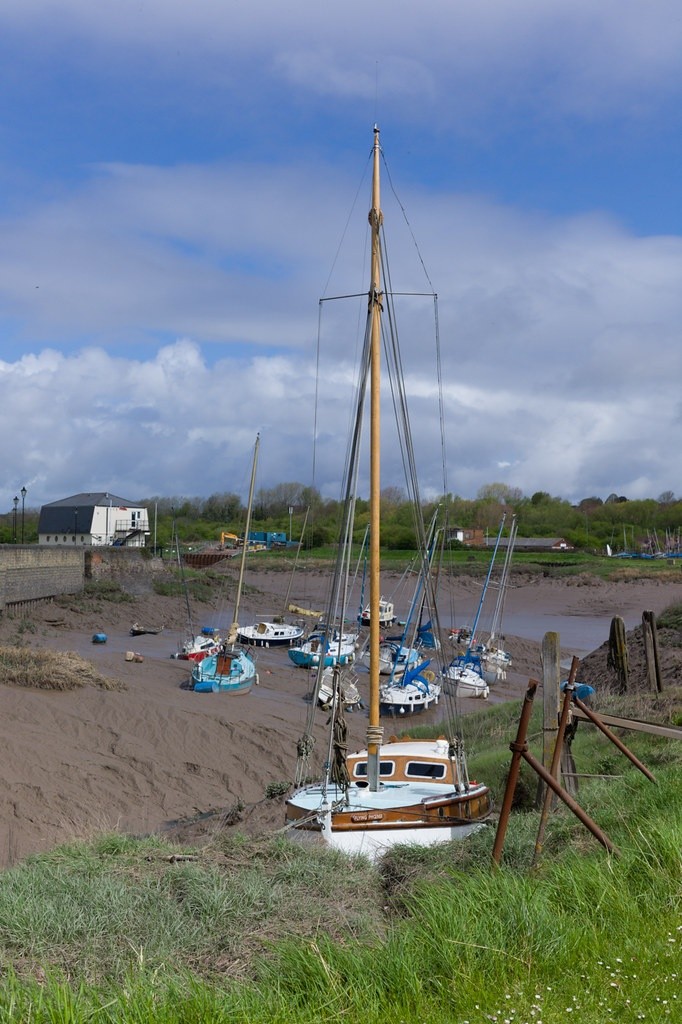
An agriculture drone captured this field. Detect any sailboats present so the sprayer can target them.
[604,523,682,562]
[442,511,508,699]
[361,527,448,674]
[189,433,260,695]
[378,504,442,716]
[283,123,492,858]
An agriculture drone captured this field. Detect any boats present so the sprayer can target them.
[287,638,357,668]
[356,600,398,628]
[236,618,303,647]
[173,634,221,664]
[314,670,361,712]
[307,628,357,647]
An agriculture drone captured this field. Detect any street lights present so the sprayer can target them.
[13,496,19,540]
[20,486,27,543]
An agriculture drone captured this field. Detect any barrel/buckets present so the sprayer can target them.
[93,634,107,642]
[559,681,596,700]
[202,626,214,634]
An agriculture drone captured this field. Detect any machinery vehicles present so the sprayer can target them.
[219,532,267,554]
